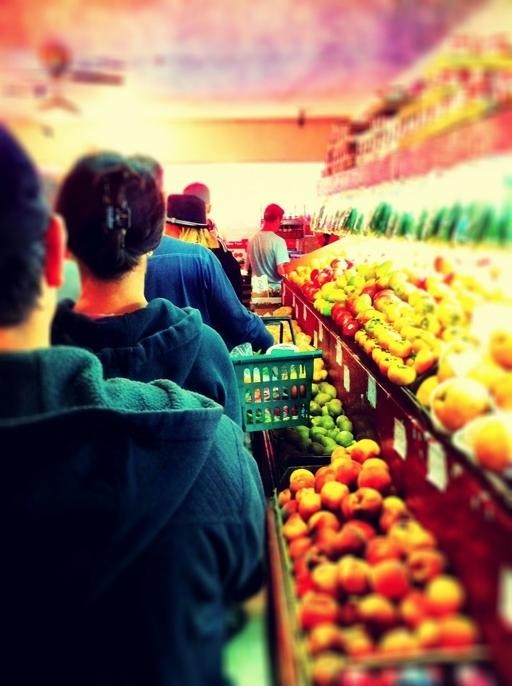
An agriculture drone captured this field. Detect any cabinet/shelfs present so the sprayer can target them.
[242,99,509,686]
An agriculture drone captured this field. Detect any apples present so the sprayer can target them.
[248,330,512,686]
[301,258,412,336]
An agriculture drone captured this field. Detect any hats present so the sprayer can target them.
[166,194,208,228]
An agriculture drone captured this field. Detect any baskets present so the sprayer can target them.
[229,349,323,432]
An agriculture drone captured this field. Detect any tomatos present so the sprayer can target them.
[356,265,512,384]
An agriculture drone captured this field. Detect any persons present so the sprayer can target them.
[128,152,276,357]
[0,119,266,686]
[163,182,242,298]
[244,204,291,296]
[49,151,242,427]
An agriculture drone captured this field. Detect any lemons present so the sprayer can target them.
[288,245,346,287]
[264,307,311,352]
